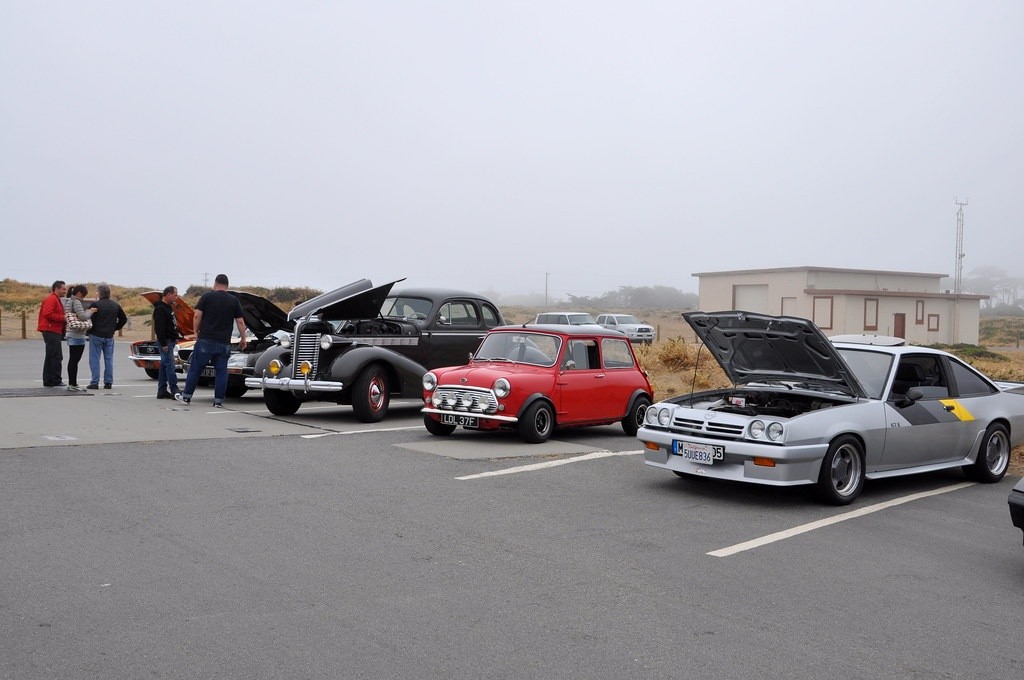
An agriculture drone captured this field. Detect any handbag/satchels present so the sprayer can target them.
[66,313,93,330]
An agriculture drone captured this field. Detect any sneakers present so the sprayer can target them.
[174,393,190,405]
[157,391,172,399]
[87,384,98,389]
[214,402,223,407]
[104,383,111,389]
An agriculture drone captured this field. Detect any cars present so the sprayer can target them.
[128,292,195,379]
[421,326,653,440]
[178,289,294,396]
[245,276,505,421]
[637,310,1023,504]
[597,313,656,342]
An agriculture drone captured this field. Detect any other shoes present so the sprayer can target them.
[67,385,87,392]
[59,381,66,386]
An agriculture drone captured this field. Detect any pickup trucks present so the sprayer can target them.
[495,310,628,338]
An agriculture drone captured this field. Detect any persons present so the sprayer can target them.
[38,281,68,387]
[87,284,127,389]
[174,274,247,407]
[64,285,98,392]
[153,286,183,400]
